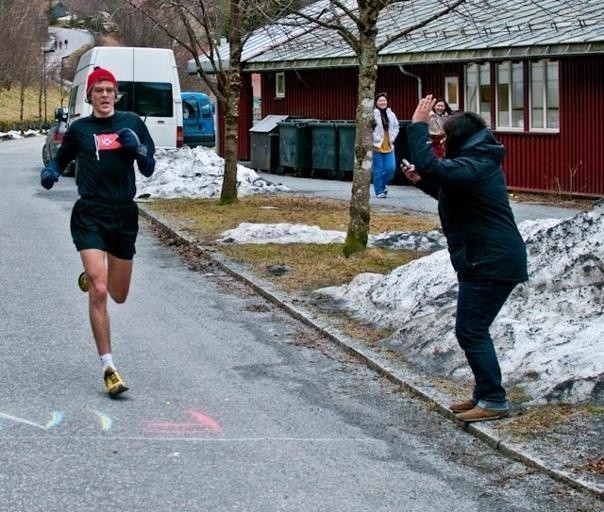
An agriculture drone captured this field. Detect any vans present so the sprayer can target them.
[179,86,217,148]
[64,46,182,151]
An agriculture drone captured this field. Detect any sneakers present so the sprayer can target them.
[105,367,130,398]
[449,398,510,423]
[78,271,89,292]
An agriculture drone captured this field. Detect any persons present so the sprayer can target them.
[372,92,399,198]
[40,66,155,397]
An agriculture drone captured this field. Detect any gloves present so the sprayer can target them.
[117,127,147,158]
[40,167,59,190]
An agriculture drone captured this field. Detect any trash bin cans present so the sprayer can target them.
[249,115,412,178]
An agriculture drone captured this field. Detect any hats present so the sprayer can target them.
[87,67,119,94]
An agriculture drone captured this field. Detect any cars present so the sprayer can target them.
[40,106,75,178]
